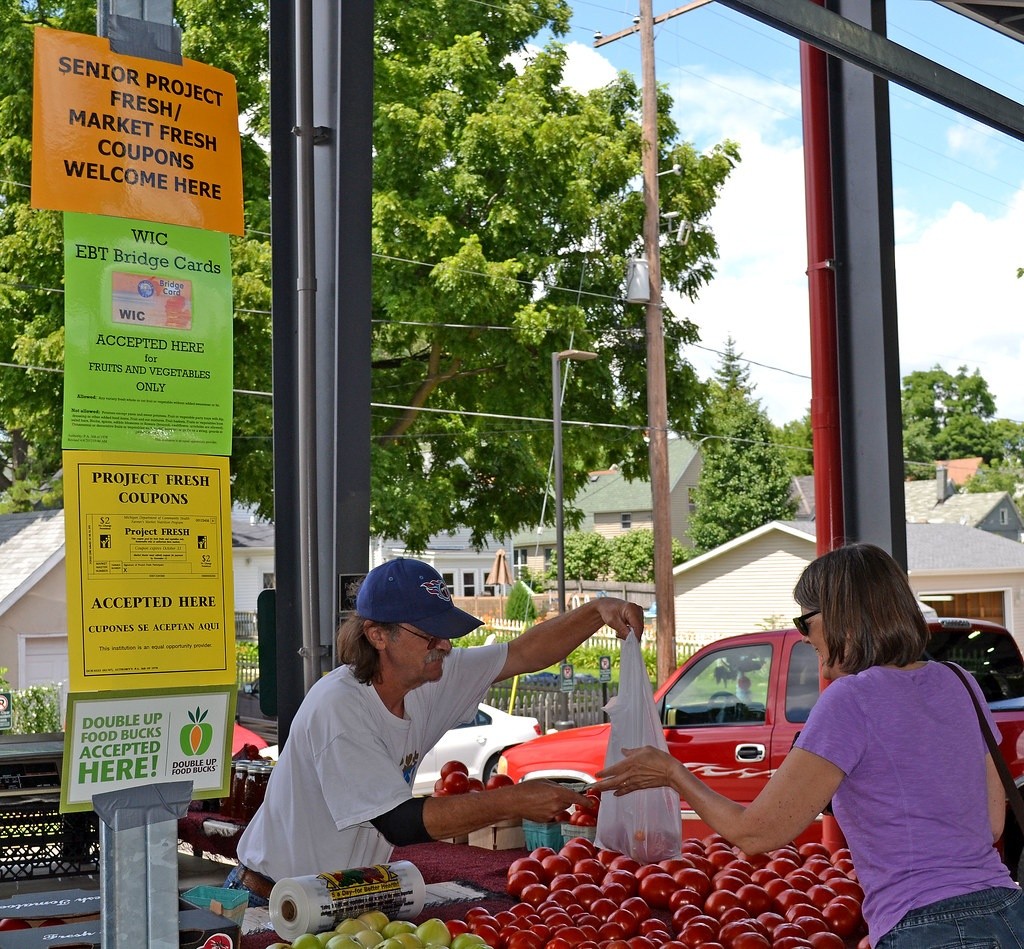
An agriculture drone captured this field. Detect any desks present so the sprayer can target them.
[178,807,536,945]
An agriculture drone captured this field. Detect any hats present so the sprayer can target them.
[358,557,485,639]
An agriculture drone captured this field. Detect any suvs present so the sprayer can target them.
[495,615,1024,813]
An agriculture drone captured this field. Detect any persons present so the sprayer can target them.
[225,557,644,910]
[587,544,1024,949]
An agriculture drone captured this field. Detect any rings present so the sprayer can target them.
[622,781,629,787]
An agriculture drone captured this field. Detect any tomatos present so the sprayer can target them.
[262,823,872,949]
[431,761,600,826]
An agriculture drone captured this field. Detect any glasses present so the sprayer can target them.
[793,610,821,636]
[394,622,449,649]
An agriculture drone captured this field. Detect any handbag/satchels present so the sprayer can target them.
[592,627,683,861]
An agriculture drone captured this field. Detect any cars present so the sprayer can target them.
[258,699,542,796]
